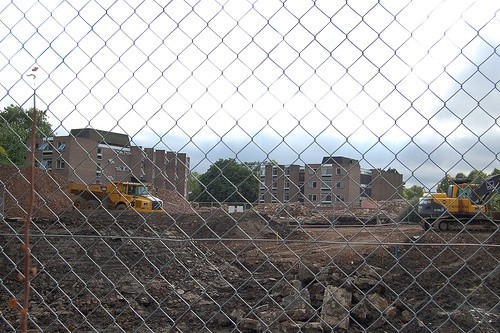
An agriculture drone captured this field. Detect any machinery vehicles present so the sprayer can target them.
[71,179,163,212]
[418,173,500,232]
